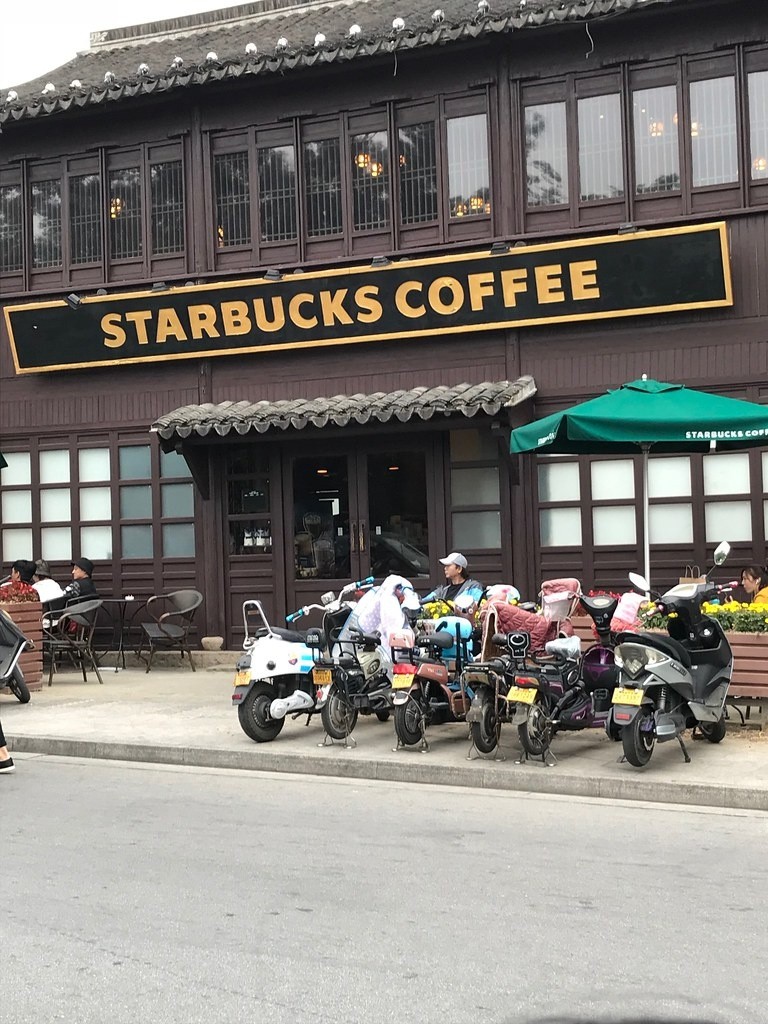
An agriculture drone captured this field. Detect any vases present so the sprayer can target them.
[0,602,43,695]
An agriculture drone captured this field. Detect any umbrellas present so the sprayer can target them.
[511,373,768,601]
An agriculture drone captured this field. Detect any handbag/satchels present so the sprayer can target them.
[679,565,706,584]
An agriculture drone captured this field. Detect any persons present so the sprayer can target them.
[724,565,768,604]
[32,559,64,628]
[64,557,96,605]
[423,552,484,607]
[0,560,37,585]
[0,721,15,774]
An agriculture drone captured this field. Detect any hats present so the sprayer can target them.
[71,557,95,578]
[34,559,51,576]
[438,552,467,569]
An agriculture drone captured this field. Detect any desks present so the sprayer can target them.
[98,598,155,673]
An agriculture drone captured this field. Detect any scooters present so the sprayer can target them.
[230,575,375,743]
[504,591,647,769]
[305,574,423,749]
[390,584,523,755]
[458,602,575,763]
[611,540,739,766]
[0,609,37,704]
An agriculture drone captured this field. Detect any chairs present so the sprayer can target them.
[137,590,203,672]
[39,593,103,685]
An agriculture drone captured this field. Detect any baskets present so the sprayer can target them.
[417,619,436,635]
[591,615,647,641]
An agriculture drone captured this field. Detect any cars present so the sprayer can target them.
[334,531,429,579]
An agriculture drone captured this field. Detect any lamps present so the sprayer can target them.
[490,242,510,254]
[618,225,637,235]
[150,281,169,293]
[387,457,401,471]
[63,294,83,309]
[371,256,391,266]
[263,270,283,280]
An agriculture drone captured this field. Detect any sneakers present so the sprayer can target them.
[0,757,15,772]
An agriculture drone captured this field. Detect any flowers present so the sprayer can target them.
[0,580,40,602]
[418,587,768,633]
